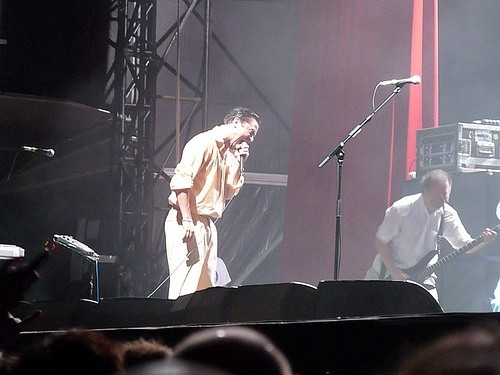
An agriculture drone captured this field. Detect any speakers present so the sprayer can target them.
[16,279,444,332]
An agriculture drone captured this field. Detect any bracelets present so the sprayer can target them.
[182,219,193,222]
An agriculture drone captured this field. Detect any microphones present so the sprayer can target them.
[23,146,55,157]
[380,75,421,86]
[239,142,248,180]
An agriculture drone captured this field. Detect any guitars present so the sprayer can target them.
[386,224,500,291]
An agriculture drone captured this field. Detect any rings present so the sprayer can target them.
[492,238,495,241]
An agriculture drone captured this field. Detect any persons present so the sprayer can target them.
[364,169,498,303]
[165,108,261,299]
[0,310,292,375]
